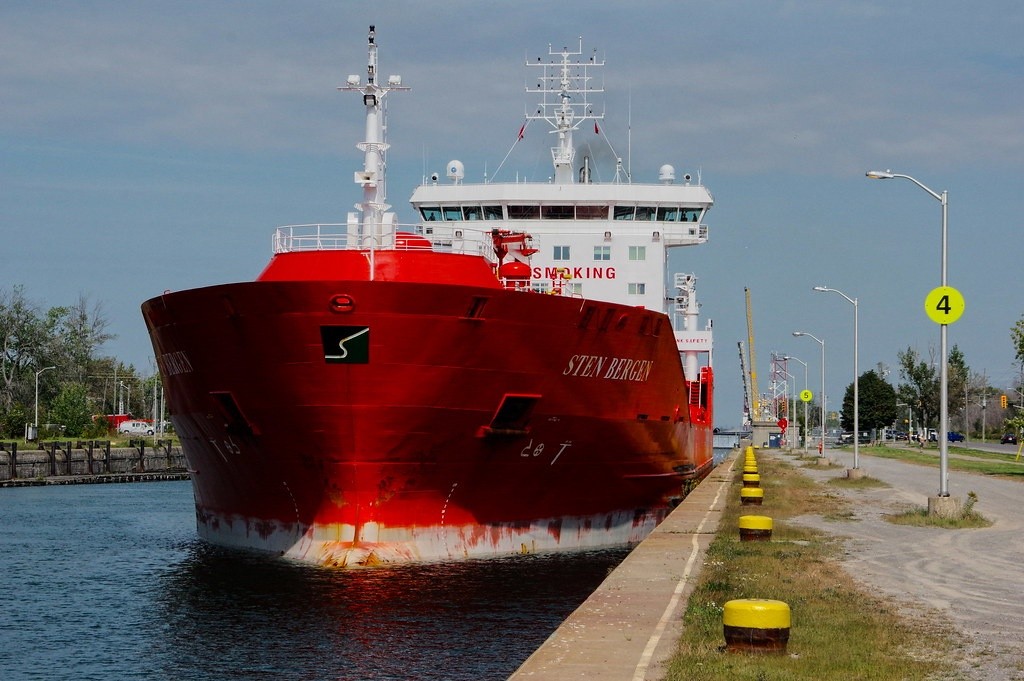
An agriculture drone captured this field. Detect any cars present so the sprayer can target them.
[1018,433,1020,444]
[834,428,964,445]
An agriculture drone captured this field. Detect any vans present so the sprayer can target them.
[119,420,155,436]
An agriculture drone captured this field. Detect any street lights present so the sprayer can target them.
[866,166,962,517]
[33,365,58,427]
[812,283,867,482]
[792,330,829,466]
[767,368,800,455]
[778,355,813,457]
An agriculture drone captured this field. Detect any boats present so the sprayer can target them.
[137,22,716,574]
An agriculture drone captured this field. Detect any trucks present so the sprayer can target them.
[91,413,133,432]
